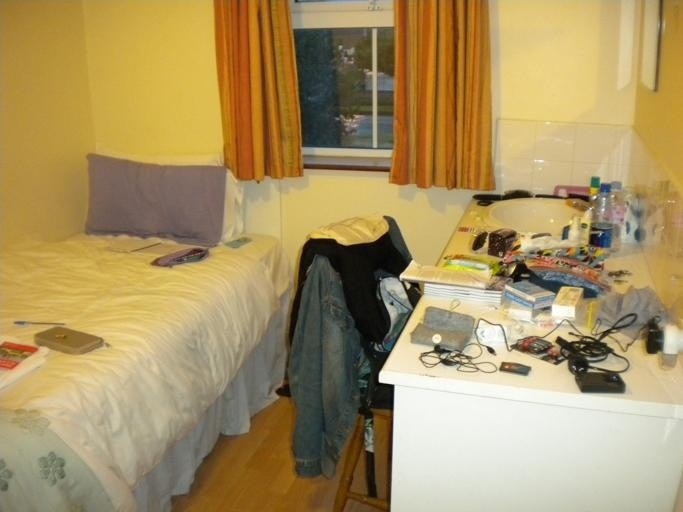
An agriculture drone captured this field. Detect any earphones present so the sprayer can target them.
[434,345,448,353]
[442,360,458,366]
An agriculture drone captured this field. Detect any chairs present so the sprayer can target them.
[286,208,416,512]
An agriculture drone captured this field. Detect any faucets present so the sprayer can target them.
[565,194,610,219]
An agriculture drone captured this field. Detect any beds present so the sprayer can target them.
[0,146,292,512]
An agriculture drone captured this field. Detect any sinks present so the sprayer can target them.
[482,197,585,236]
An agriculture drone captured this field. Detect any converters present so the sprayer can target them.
[646,322,664,354]
[576,372,625,393]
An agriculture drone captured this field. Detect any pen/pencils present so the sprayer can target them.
[14,321,65,325]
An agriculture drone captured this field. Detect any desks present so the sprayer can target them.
[375,174,681,512]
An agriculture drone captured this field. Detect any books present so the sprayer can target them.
[0,336,50,394]
[398,259,556,310]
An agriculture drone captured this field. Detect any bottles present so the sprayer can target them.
[588,183,613,257]
[609,181,624,254]
[589,177,601,197]
[569,206,596,246]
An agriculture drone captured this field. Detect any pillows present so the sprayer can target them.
[80,150,230,248]
[108,151,245,244]
